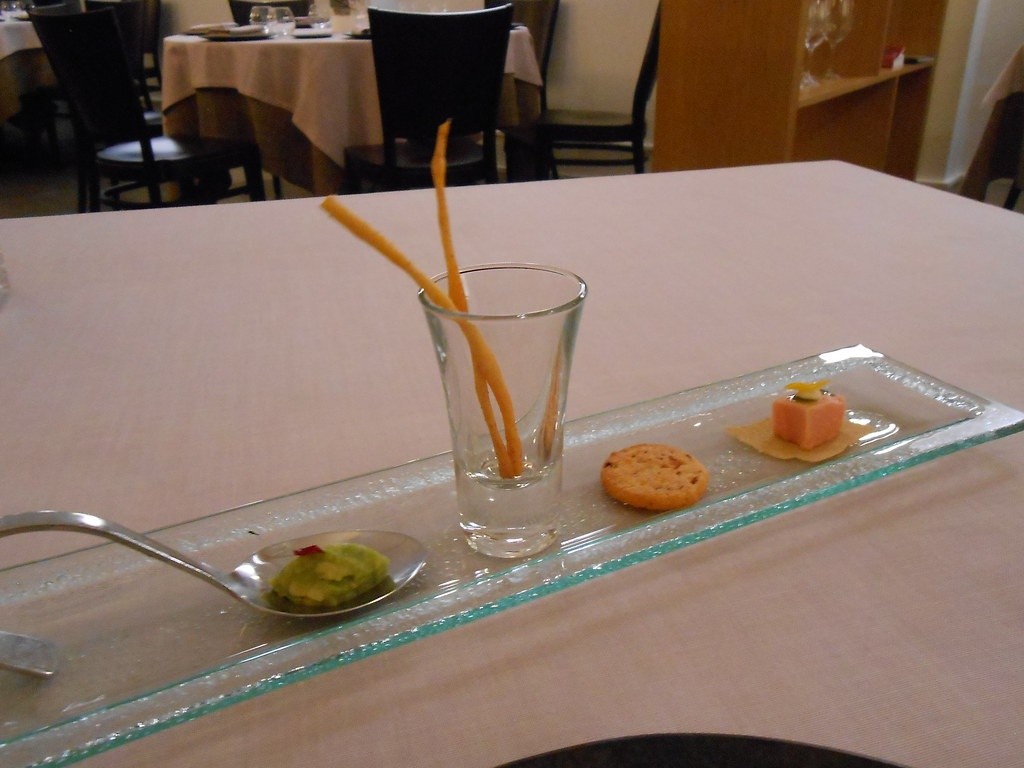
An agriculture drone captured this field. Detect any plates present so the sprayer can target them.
[185,22,372,40]
[1,339,1024,768]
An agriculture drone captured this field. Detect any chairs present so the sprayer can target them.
[28,1,667,210]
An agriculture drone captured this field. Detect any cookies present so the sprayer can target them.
[599,444,709,512]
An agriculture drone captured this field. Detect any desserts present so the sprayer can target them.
[725,378,873,462]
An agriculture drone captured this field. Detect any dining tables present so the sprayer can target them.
[1,140,1024,768]
[0,7,62,166]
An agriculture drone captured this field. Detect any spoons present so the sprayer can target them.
[0,508,427,621]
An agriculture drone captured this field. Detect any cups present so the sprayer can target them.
[249,5,274,28]
[307,4,335,30]
[0,0,34,20]
[418,261,590,561]
[266,6,295,40]
